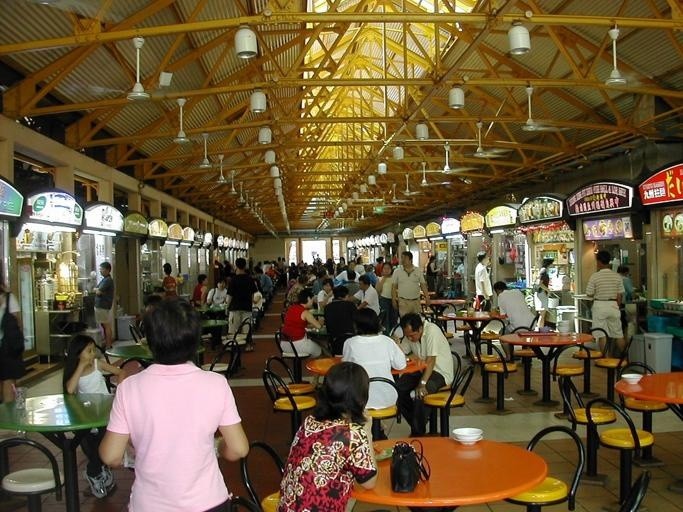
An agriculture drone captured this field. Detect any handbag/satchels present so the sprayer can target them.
[390,439,431,493]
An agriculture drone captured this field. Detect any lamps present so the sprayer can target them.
[414,121,428,143]
[316,141,486,238]
[507,21,531,56]
[233,22,258,60]
[248,88,266,114]
[447,85,465,110]
[258,125,272,144]
[201,149,295,245]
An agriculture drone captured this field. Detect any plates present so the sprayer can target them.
[454,311,463,316]
[371,447,392,461]
[661,212,683,234]
[453,438,482,446]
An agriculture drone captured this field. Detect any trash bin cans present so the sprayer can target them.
[644,333,674,374]
[117,316,136,340]
[627,333,644,373]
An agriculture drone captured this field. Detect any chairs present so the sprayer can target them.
[236,442,323,511]
[620,362,667,465]
[596,468,653,512]
[0,434,65,512]
[587,398,657,512]
[503,424,583,512]
[225,494,262,512]
[554,376,618,477]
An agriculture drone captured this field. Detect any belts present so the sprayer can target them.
[399,297,417,300]
[594,299,618,301]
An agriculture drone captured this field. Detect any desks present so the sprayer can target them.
[349,435,549,512]
[0,395,123,512]
[613,365,682,492]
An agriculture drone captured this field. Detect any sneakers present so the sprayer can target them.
[100,464,113,487]
[245,343,254,351]
[85,470,108,499]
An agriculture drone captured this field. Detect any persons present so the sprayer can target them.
[0,276,26,405]
[93,262,116,353]
[280,249,455,439]
[63,335,127,396]
[161,255,287,335]
[474,249,639,367]
[277,360,413,512]
[96,296,250,512]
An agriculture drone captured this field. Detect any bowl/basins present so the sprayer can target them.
[451,426,483,447]
[556,320,570,336]
[621,372,644,385]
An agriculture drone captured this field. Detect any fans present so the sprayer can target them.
[68,36,186,103]
[148,98,221,151]
[504,82,574,134]
[577,22,661,91]
[461,117,515,162]
[178,127,234,172]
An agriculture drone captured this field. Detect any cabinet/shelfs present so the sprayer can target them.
[34,308,72,364]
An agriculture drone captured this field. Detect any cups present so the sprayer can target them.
[466,307,473,317]
[12,386,28,411]
